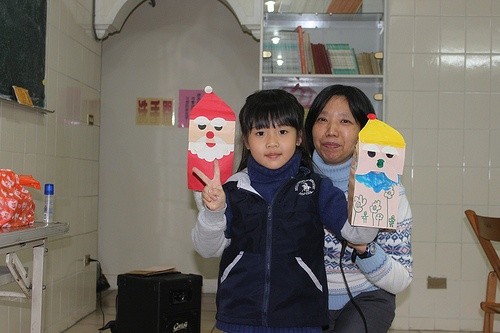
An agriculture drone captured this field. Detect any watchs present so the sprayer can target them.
[350,241,376,263]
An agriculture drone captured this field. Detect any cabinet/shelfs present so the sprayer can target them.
[260,0,387,122]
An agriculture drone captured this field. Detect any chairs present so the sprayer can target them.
[465,210,500,333]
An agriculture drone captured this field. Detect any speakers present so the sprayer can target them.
[115,274,202,333]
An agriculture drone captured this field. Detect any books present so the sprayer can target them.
[260,1,384,76]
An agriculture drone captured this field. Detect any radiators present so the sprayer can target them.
[0,262,97,333]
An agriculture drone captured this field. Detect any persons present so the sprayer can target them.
[307,85,413,333]
[190,91,391,333]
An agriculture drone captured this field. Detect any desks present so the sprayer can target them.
[0,220,69,333]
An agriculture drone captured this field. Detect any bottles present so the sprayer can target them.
[42,183,55,224]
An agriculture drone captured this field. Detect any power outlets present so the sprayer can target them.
[84,254,90,265]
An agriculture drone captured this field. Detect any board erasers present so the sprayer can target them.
[11,85,33,106]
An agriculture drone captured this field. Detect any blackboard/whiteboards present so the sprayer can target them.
[0,0,56,115]
